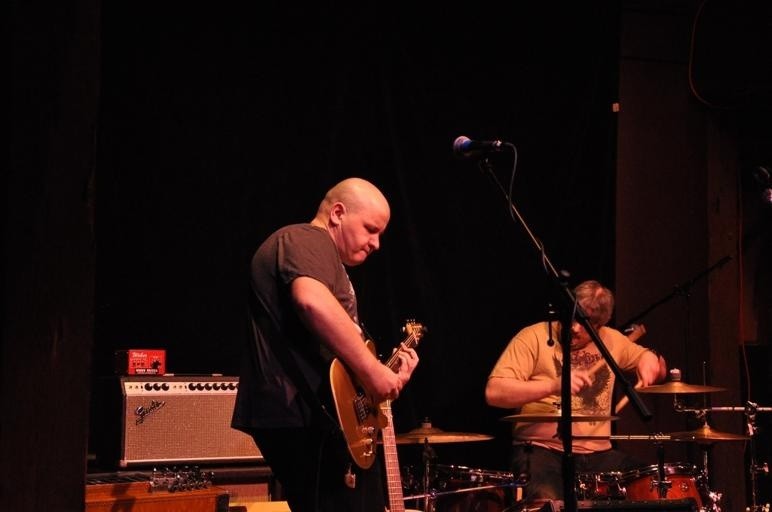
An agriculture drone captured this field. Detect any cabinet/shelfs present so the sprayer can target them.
[83,467,290,512]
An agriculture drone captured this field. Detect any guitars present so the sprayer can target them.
[303,318,426,475]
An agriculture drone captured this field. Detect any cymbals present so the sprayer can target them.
[666,425,747,442]
[504,406,618,423]
[628,382,727,396]
[375,426,493,445]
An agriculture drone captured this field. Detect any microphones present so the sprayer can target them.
[452,135,503,156]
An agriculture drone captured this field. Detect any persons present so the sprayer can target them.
[225,174,421,511]
[482,276,660,512]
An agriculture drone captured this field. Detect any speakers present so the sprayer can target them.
[108,374,265,469]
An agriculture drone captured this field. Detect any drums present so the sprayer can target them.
[577,470,623,498]
[432,464,525,512]
[620,462,706,512]
